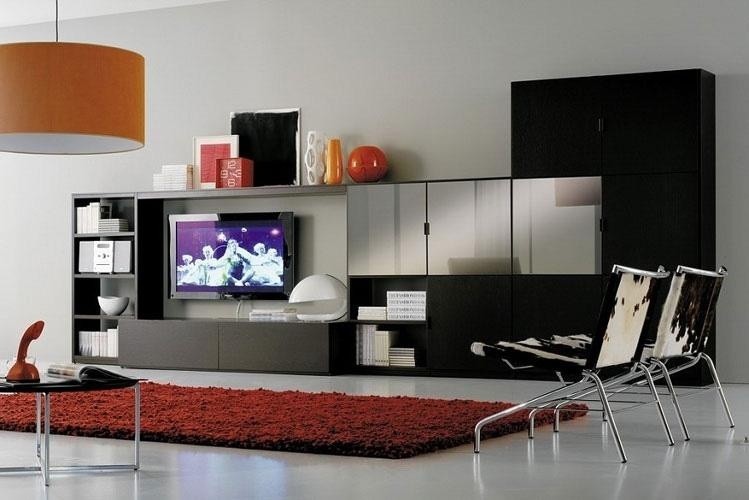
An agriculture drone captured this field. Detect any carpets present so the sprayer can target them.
[0,382,588,458]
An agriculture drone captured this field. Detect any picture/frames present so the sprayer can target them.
[192,135,239,189]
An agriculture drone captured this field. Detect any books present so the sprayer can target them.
[153,163,193,192]
[79,323,119,359]
[76,200,129,235]
[355,289,427,371]
[0,362,150,389]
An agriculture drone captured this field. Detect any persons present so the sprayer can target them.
[176,239,283,287]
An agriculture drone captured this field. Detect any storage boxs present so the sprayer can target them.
[215,158,253,188]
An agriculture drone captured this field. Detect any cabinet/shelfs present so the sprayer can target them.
[67,192,164,365]
[218,317,332,376]
[426,275,512,380]
[509,70,715,387]
[332,274,428,377]
[116,318,219,372]
[345,177,511,278]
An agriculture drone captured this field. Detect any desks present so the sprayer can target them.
[0,377,148,488]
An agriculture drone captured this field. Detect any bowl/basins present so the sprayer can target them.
[98,295,130,317]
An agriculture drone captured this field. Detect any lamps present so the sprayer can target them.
[0,0,146,155]
[288,274,348,322]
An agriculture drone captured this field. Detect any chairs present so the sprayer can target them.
[546,265,735,441]
[468,265,676,464]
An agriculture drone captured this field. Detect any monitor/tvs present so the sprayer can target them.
[167,211,295,300]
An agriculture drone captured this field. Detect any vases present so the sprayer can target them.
[305,131,325,186]
[346,146,389,183]
[324,139,343,185]
